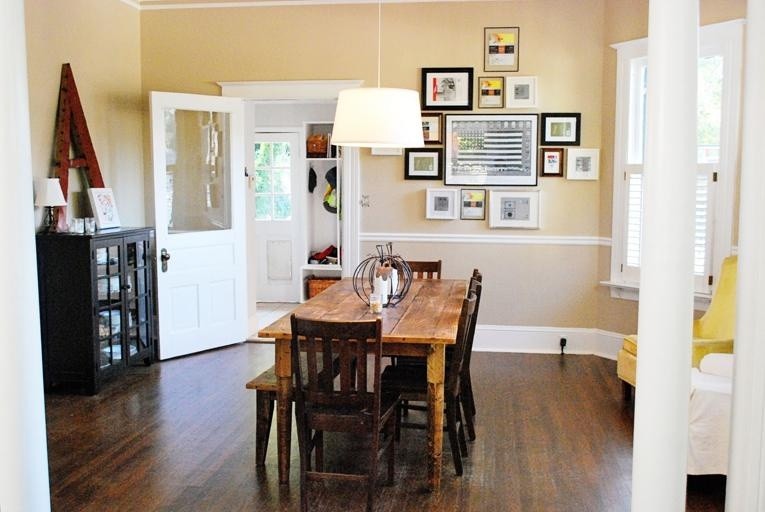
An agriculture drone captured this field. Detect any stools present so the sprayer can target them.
[688,353,735,480]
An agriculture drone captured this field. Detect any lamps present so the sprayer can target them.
[33,178,69,232]
[329,1,426,153]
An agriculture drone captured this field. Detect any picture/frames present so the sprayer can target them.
[541,148,564,176]
[87,186,121,231]
[506,76,539,108]
[488,190,539,230]
[425,187,457,219]
[478,76,504,108]
[540,112,582,147]
[483,27,520,72]
[567,148,600,181]
[420,68,473,110]
[421,112,443,145]
[460,188,486,220]
[407,147,443,180]
[444,113,538,187]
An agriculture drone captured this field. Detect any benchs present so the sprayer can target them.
[246,351,362,466]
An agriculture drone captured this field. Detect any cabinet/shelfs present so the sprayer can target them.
[299,267,343,304]
[36,225,156,397]
[302,119,342,269]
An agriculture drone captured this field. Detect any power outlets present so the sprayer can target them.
[558,335,568,349]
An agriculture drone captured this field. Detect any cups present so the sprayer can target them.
[369,294,385,313]
[69,217,96,233]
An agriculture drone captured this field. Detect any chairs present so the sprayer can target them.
[371,292,481,473]
[616,252,742,404]
[380,256,442,365]
[473,267,484,285]
[285,312,402,511]
[381,278,481,441]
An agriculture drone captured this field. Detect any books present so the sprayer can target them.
[87,187,121,230]
